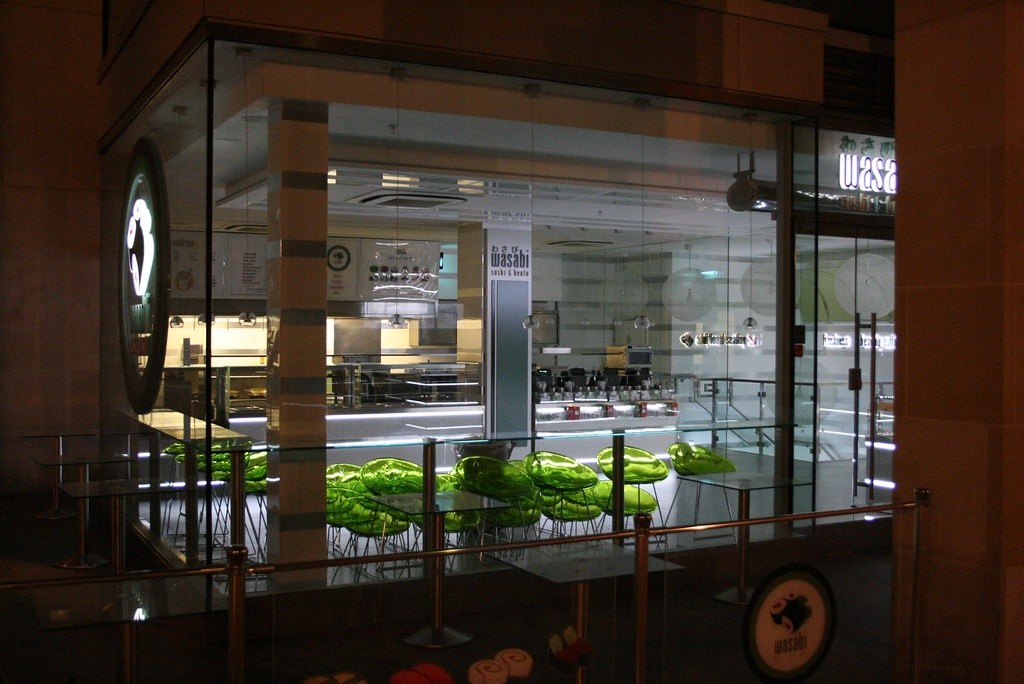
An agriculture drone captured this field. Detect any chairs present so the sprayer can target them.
[155,435,739,591]
